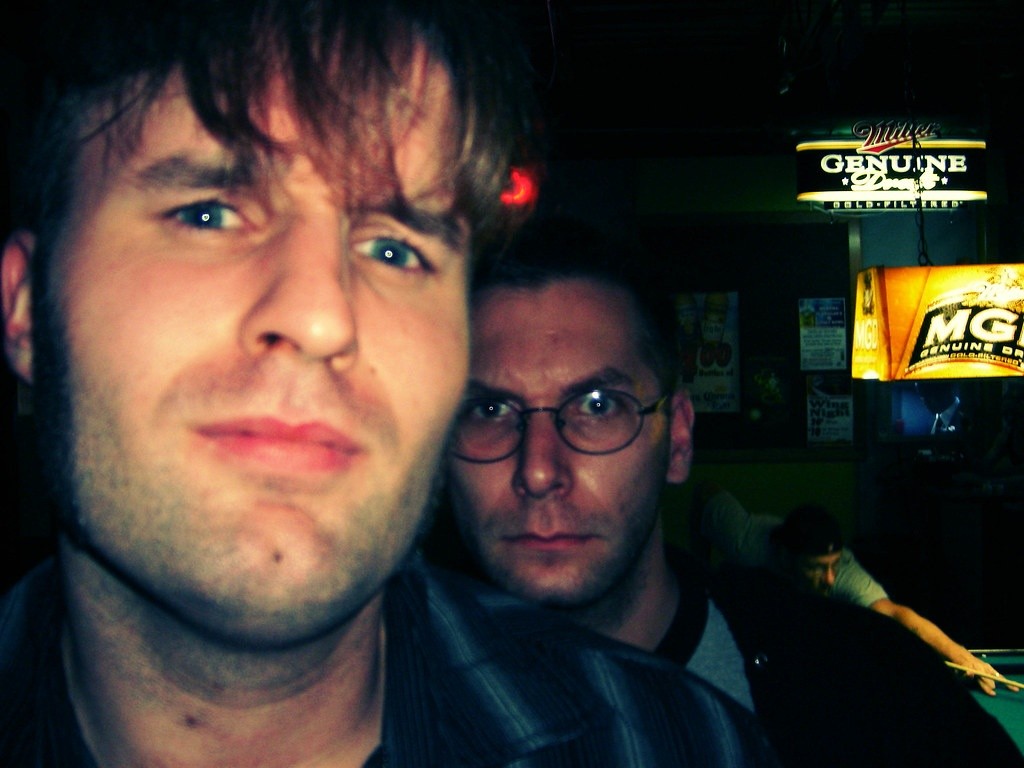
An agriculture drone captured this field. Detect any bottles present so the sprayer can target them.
[671,293,730,353]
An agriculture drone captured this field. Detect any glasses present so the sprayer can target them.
[445,385,673,464]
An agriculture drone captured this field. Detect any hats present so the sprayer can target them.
[770,502,844,555]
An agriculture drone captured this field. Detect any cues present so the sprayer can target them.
[944,660,1024,688]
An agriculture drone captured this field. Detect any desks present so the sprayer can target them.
[940,648,1024,768]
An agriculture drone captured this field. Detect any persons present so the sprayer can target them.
[0,0,784,768]
[426,211,1022,768]
[686,455,1020,697]
[980,386,1023,493]
